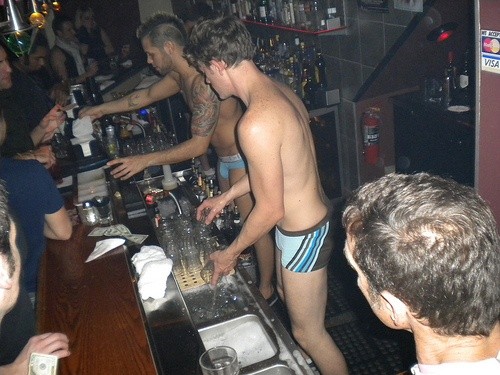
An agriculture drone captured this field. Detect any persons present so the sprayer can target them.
[47,0,116,117]
[182,13,348,375]
[77,12,279,308]
[342,171,500,375]
[0,30,73,375]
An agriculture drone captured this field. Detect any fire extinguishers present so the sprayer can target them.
[360,107,384,164]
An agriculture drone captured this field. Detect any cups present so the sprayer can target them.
[199,346,241,375]
[159,208,230,284]
[63,195,114,229]
[423,79,443,104]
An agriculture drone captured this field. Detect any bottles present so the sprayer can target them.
[180,1,344,32]
[251,33,326,110]
[181,155,252,255]
[106,108,180,158]
[138,164,179,230]
[442,47,473,107]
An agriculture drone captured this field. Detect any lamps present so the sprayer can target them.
[1,0,38,58]
[29,0,61,28]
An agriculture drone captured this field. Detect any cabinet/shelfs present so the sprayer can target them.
[307,106,343,209]
[389,88,477,188]
